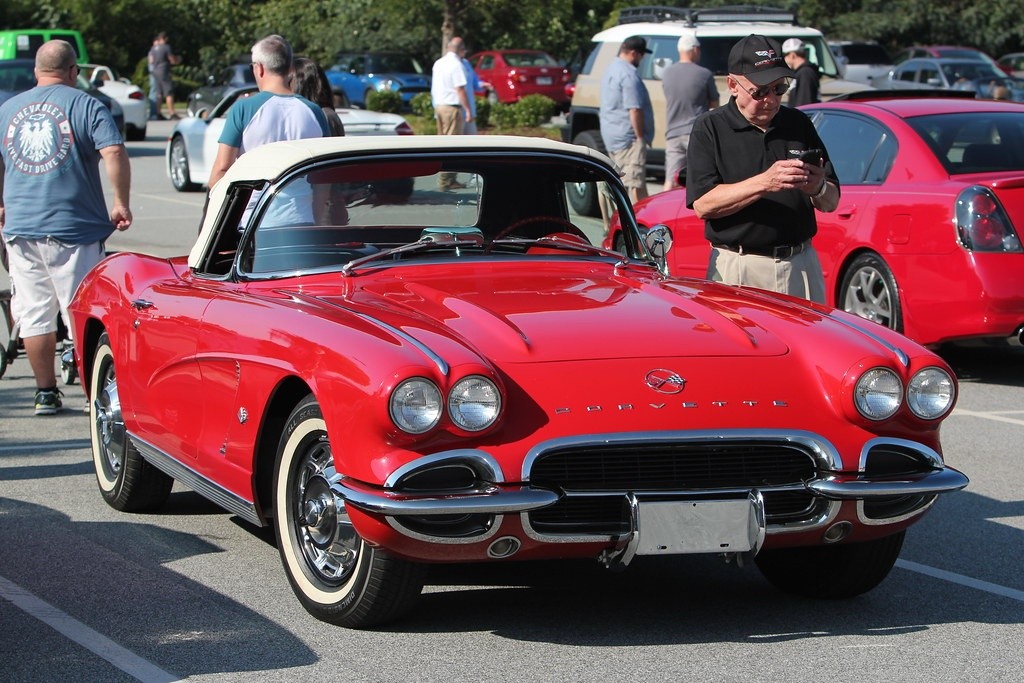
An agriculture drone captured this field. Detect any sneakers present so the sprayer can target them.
[34,390,64,414]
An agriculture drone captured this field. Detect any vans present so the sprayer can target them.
[0,29,88,78]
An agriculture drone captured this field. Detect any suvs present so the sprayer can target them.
[559,8,878,217]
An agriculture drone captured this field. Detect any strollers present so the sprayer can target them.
[0,233,120,386]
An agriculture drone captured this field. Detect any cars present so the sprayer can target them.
[165,58,415,192]
[467,51,573,116]
[323,48,433,114]
[602,40,1024,351]
[0,60,148,141]
[67,134,969,629]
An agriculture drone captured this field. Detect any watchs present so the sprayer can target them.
[810,178,828,200]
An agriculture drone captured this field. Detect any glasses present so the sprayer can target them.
[733,76,791,100]
[70,64,80,75]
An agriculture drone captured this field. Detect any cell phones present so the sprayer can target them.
[799,149,823,168]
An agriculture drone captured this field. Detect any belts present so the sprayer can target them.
[710,237,812,260]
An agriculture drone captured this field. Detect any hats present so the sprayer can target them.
[728,35,800,86]
[625,35,652,54]
[780,37,813,57]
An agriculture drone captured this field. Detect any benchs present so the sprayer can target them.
[217,226,543,275]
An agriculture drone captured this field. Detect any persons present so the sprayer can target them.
[661,33,720,190]
[685,34,841,307]
[0,40,133,415]
[288,57,344,137]
[431,37,494,135]
[993,79,1007,99]
[953,70,977,90]
[198,35,330,231]
[598,35,654,205]
[148,30,181,120]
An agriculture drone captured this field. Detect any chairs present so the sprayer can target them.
[961,142,1009,165]
